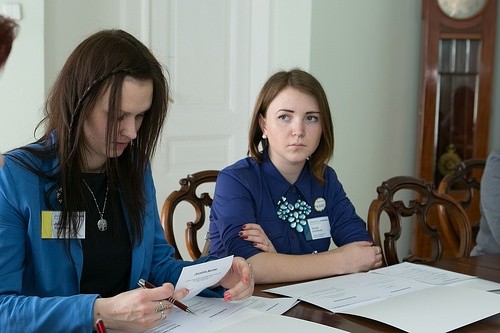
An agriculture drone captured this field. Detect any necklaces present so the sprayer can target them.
[82,179,108,232]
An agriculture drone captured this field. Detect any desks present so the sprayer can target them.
[253,252,500,333]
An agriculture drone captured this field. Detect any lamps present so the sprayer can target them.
[411,0,498,259]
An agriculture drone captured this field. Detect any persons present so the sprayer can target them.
[210,70,382,283]
[471,149,500,256]
[0,29,255,333]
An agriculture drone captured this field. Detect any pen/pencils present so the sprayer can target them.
[95,319,107,333]
[137,279,194,315]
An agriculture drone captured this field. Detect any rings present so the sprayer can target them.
[161,311,166,320]
[156,301,168,312]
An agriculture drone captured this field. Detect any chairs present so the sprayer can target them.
[366,174,472,268]
[160,169,220,261]
[436,158,485,258]
